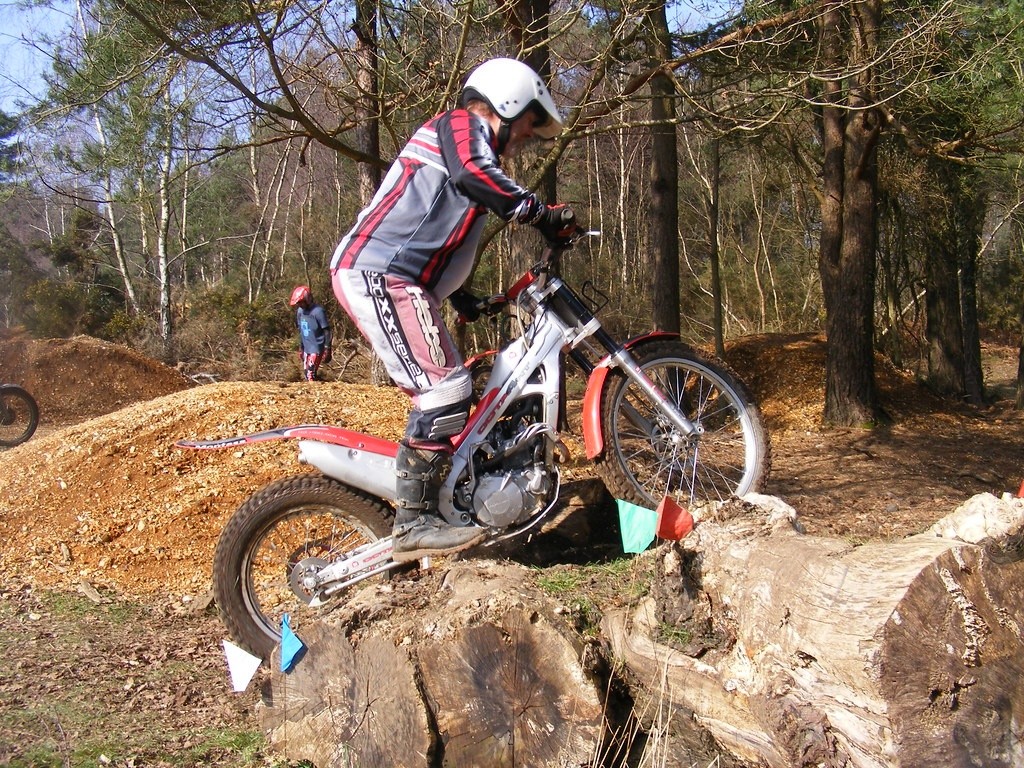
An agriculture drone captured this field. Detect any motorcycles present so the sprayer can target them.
[168,204,775,676]
[0,383,40,448]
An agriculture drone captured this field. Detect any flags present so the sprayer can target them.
[221,615,303,693]
[613,494,695,551]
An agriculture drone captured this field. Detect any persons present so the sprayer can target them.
[330,57,580,561]
[290,285,333,382]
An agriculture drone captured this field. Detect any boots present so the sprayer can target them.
[392,444,488,564]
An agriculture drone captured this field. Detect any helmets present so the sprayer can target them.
[463,58,563,140]
[290,285,310,307]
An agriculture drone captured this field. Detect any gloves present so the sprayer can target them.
[299,348,304,363]
[321,347,332,364]
[521,201,584,250]
[447,288,481,321]
